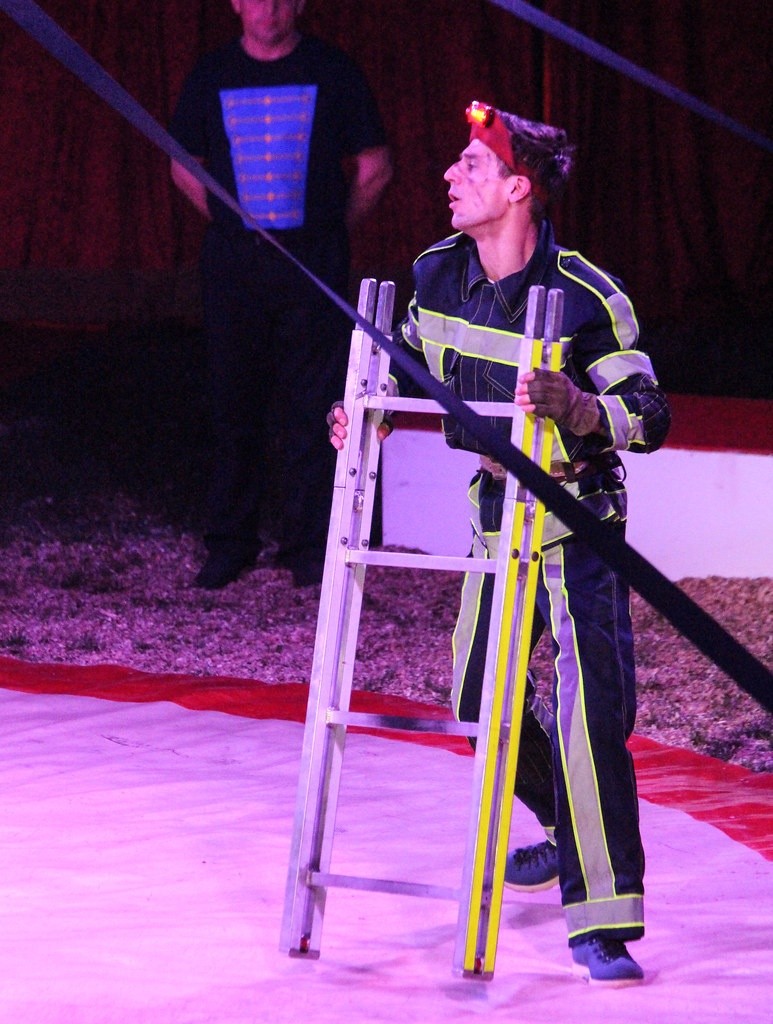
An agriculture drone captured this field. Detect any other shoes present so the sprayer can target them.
[293,561,324,587]
[189,563,241,589]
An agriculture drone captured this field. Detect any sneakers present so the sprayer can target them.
[502,839,559,892]
[572,936,645,989]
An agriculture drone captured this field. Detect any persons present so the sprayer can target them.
[163,0,394,584]
[327,100,670,989]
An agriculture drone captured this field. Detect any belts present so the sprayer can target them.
[479,452,610,485]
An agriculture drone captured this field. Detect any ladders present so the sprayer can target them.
[279,280,566,982]
